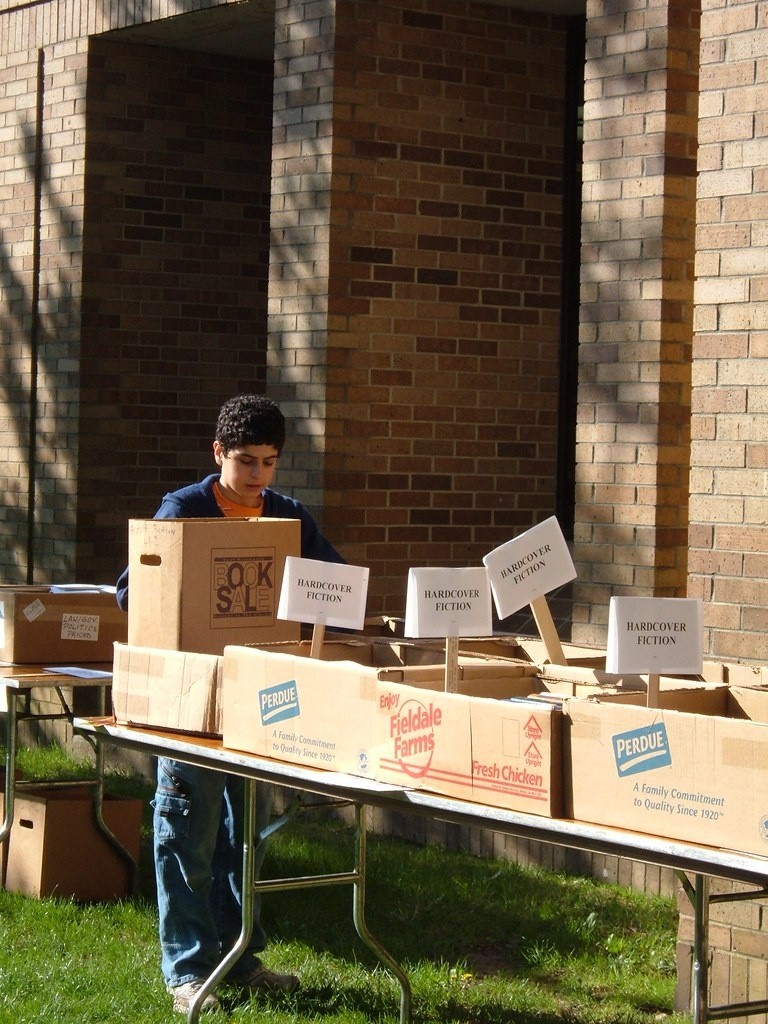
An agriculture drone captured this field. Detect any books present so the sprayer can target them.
[499,692,575,710]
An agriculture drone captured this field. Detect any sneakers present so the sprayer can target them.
[167,981,219,1014]
[215,964,300,1002]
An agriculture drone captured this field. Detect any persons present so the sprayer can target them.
[117,396,349,1013]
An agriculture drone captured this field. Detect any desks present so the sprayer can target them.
[66,713,767,1024]
[0,661,139,902]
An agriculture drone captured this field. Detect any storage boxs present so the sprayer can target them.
[0,583,128,663]
[0,764,144,902]
[111,516,766,859]
